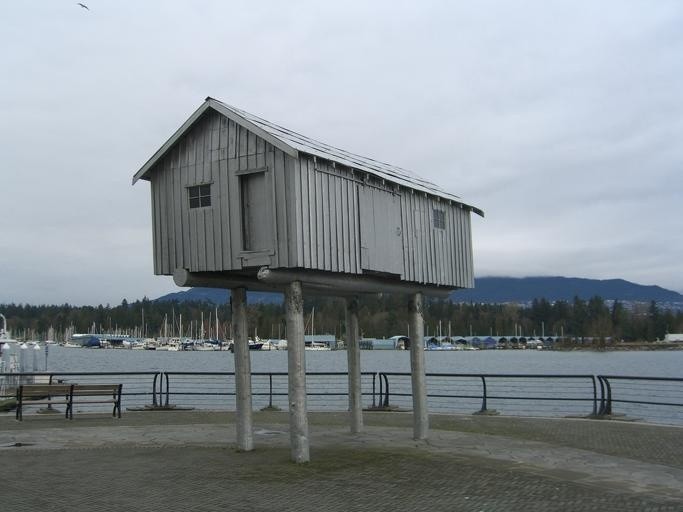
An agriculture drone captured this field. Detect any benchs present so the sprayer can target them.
[15,383,123,421]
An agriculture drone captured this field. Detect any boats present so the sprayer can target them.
[1,352,57,412]
